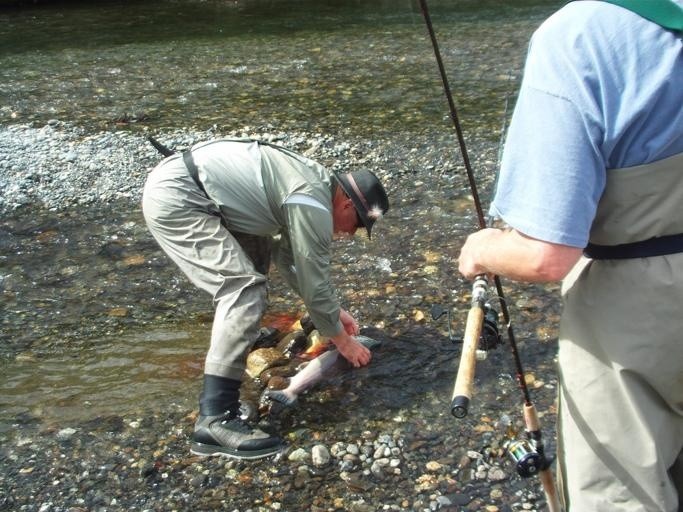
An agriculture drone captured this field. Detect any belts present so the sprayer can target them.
[182,148,211,199]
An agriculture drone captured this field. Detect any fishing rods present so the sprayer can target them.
[421,4,562,511]
[448,70,513,418]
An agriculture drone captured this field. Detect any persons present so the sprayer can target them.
[139,135,392,461]
[452,1,683,512]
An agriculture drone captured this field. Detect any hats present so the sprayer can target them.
[332,167,389,239]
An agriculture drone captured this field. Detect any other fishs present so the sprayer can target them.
[265,335,383,409]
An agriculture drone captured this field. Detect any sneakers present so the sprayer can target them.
[190,403,287,460]
